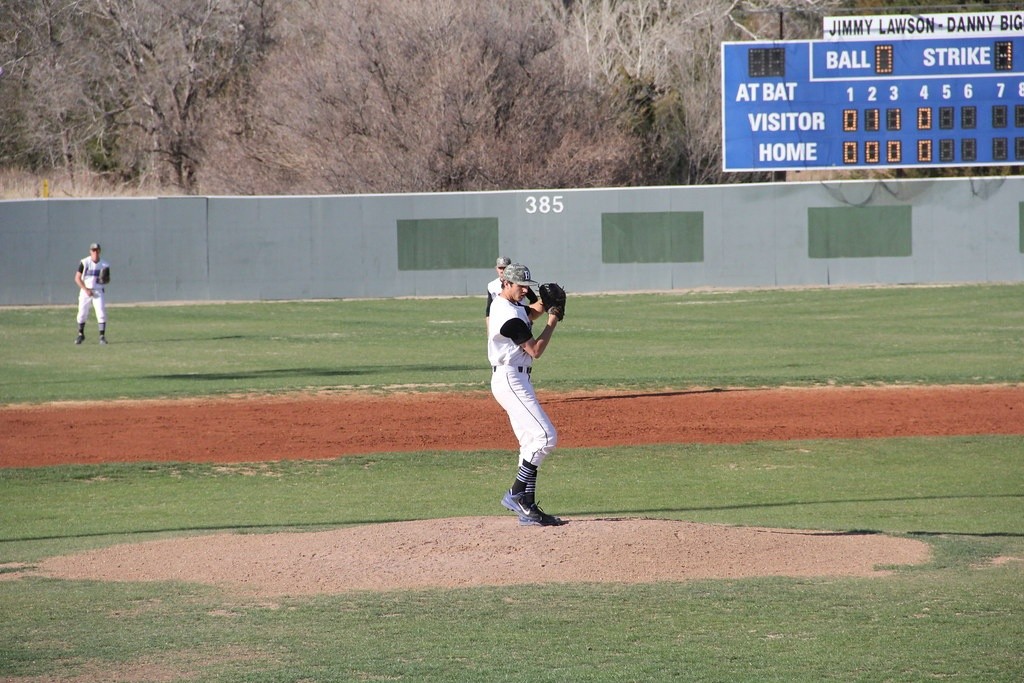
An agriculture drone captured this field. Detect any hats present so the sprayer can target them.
[503,262,538,286]
[496,257,511,267]
[90,243,100,250]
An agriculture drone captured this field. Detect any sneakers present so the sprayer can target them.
[500,489,543,520]
[519,500,564,525]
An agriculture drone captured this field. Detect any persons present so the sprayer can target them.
[486,256,538,336]
[76,243,110,344]
[488,265,569,527]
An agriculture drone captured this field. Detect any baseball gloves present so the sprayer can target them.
[99,267,110,284]
[536,281,567,321]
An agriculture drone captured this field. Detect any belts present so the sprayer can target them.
[494,366,532,373]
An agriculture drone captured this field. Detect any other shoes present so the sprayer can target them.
[100,339,108,344]
[75,334,84,344]
